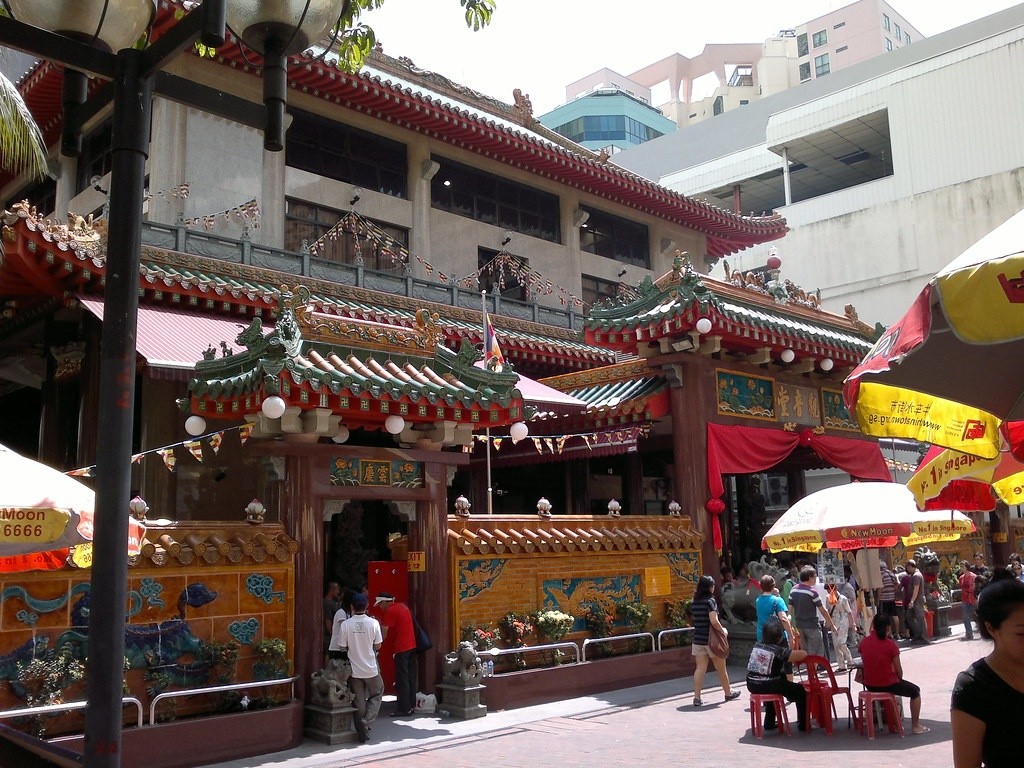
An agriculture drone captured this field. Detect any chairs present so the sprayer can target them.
[793,655,858,737]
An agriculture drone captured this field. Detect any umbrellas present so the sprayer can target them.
[0,444,146,574]
[843,206,1024,510]
[761,478,977,639]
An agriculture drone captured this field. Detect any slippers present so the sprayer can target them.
[914,726,930,733]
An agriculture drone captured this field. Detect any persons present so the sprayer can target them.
[950,565,1024,768]
[719,553,1024,734]
[690,576,740,707]
[323,581,417,744]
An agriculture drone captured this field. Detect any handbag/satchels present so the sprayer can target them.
[770,600,786,631]
[411,615,433,653]
[707,598,729,659]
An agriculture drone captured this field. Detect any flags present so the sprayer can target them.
[486,311,505,373]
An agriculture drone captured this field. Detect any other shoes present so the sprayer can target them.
[835,668,847,672]
[961,636,973,641]
[356,719,369,743]
[693,698,703,706]
[390,707,414,717]
[725,690,741,701]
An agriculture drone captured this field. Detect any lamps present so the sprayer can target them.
[781,348,795,363]
[261,392,285,420]
[510,421,528,440]
[820,357,833,371]
[184,414,206,437]
[350,186,363,205]
[330,424,349,444]
[502,229,513,245]
[385,414,405,435]
[695,317,712,334]
[617,262,630,277]
[90,175,108,196]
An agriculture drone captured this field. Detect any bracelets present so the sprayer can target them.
[911,601,913,603]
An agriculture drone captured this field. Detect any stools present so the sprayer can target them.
[748,691,792,741]
[858,690,906,740]
[798,680,839,727]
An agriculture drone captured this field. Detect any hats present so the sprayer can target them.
[374,596,395,606]
